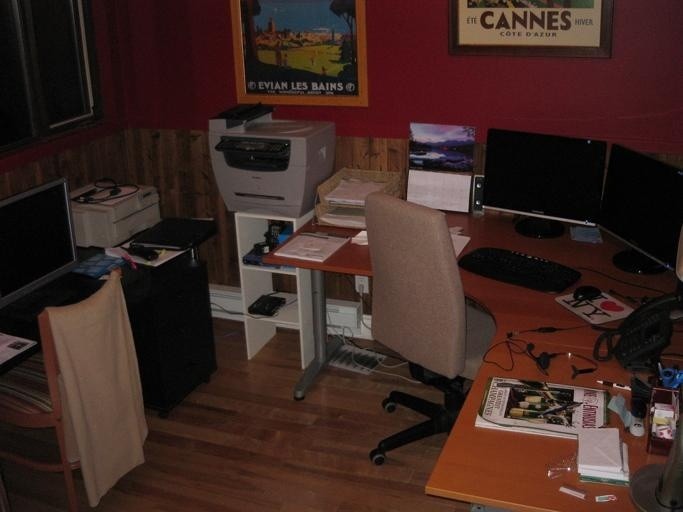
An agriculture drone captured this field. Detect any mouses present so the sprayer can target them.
[572,285,601,301]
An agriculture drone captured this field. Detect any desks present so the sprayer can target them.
[265,190,682,511]
[0,216,220,469]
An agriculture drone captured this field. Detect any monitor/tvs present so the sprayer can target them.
[598,142,683,275]
[481,127,608,240]
[0,175,79,311]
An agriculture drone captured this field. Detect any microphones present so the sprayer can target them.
[527,343,538,362]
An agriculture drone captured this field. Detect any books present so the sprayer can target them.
[577,425,631,488]
[319,178,387,229]
[273,231,349,263]
[474,375,608,439]
[103,215,219,268]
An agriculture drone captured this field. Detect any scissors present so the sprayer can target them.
[661,368,683,387]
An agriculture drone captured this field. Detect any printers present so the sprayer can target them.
[69,179,162,247]
[208,104,337,219]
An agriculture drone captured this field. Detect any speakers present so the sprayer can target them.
[470,173,485,216]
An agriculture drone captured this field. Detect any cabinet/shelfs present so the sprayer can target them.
[236,206,317,372]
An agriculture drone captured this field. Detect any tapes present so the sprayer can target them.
[630,421,644,436]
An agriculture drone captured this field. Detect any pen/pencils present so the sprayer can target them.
[596,380,632,391]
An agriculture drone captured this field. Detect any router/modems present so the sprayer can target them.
[248,294,286,316]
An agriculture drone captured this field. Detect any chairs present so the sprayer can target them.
[0,269,131,509]
[360,189,497,466]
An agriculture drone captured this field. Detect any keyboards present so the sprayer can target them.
[458,246,582,294]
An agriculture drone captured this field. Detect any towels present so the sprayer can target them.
[42,272,151,508]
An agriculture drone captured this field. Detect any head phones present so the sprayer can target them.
[538,351,598,378]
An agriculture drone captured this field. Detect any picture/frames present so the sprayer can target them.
[226,1,370,108]
[447,1,614,60]
